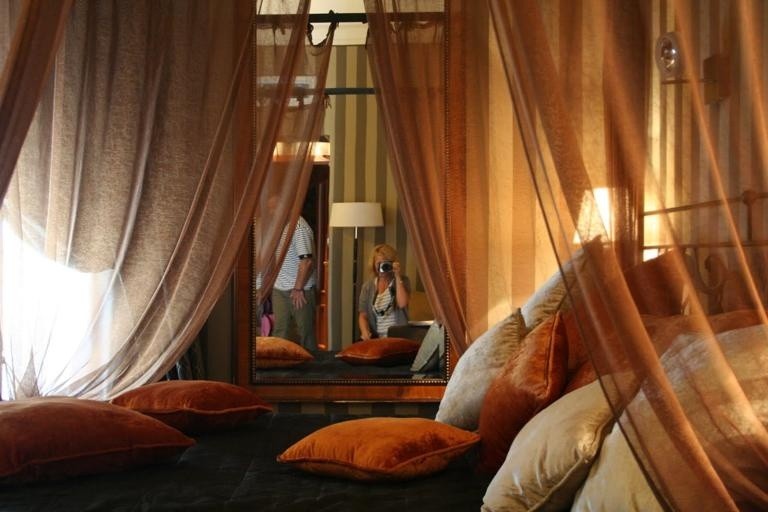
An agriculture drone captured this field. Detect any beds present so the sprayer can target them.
[1,185,767,512]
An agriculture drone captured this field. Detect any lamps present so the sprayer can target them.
[652,30,734,108]
[327,202,385,344]
[570,181,636,246]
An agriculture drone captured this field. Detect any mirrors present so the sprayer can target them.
[229,0,489,404]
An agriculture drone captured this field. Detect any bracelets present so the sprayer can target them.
[293,288,303,291]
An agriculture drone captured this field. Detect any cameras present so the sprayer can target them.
[378,260,393,272]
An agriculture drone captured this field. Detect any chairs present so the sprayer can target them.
[408,286,434,324]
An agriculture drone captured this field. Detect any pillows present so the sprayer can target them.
[255,337,312,369]
[523,235,602,333]
[481,313,568,465]
[433,308,525,431]
[1,397,192,484]
[109,381,272,429]
[335,337,420,366]
[480,371,636,512]
[276,416,480,482]
[409,319,445,373]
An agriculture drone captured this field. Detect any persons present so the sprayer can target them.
[267,194,317,350]
[358,244,411,341]
